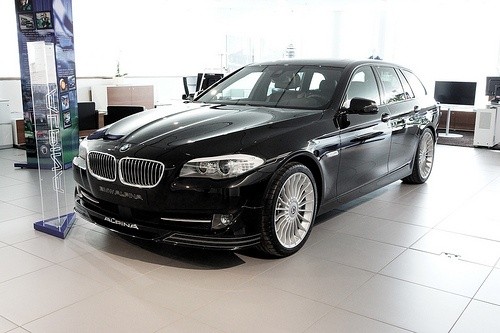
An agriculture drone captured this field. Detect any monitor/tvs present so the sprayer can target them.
[434,81,477,105]
[196,73,224,92]
[485,77,500,102]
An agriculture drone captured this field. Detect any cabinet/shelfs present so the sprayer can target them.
[106,85,154,110]
[437,111,476,131]
[0,99,12,148]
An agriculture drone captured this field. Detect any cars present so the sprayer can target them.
[72,56,442,260]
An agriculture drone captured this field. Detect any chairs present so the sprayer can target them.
[104,103,148,127]
[315,77,337,97]
[75,101,100,130]
[266,72,302,101]
